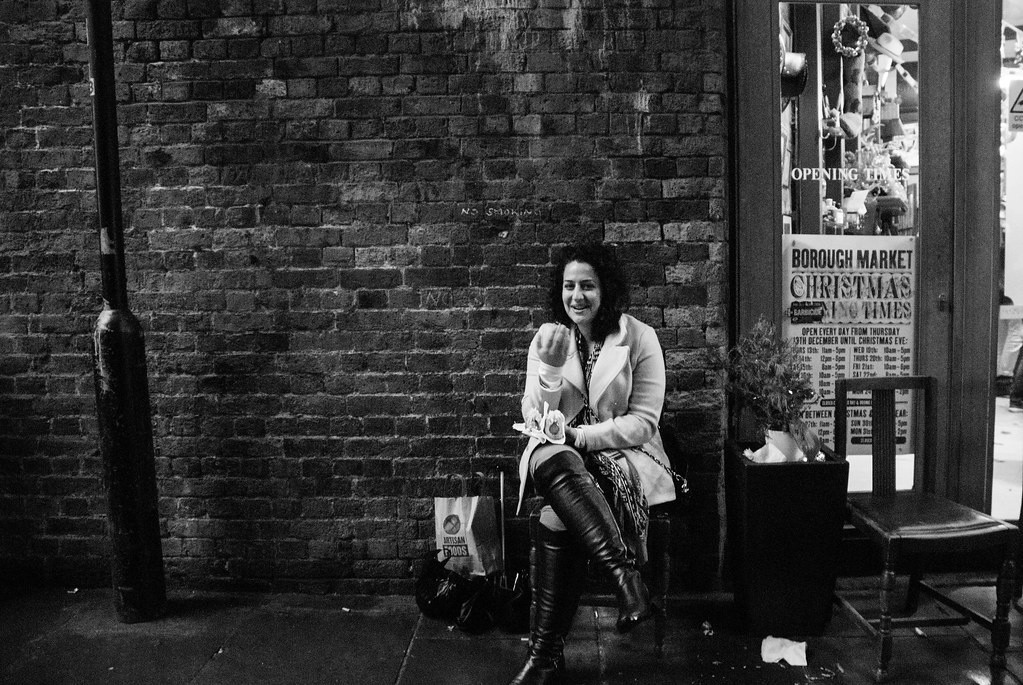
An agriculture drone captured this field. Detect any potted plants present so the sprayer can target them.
[707,313,851,633]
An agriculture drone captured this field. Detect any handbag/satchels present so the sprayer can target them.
[666,474,704,520]
[453,574,503,634]
[432,470,506,582]
[414,547,471,620]
[499,568,532,636]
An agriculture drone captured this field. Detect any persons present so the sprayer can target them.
[508,241,678,684]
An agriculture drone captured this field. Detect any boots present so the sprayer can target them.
[510,521,588,685]
[532,450,664,634]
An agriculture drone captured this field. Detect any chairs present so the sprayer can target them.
[835,376,1023,685]
[524,399,675,657]
